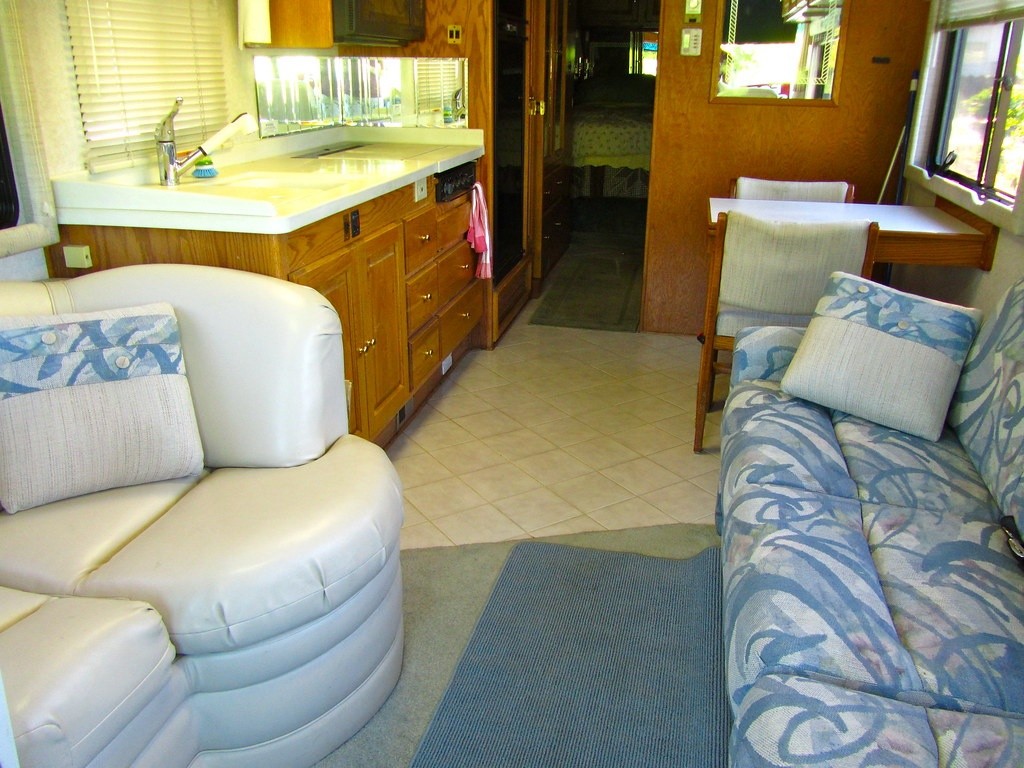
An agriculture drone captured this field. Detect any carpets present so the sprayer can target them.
[409,538,734,768]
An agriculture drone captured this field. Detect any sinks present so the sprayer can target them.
[186,171,375,193]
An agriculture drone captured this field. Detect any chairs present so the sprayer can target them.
[693,209,880,453]
[728,175,854,203]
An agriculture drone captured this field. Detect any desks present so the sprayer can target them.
[706,197,1001,275]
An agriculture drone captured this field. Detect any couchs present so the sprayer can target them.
[713,279,1024,768]
[0,262,402,768]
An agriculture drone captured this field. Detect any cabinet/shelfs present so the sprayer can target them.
[43,217,409,440]
[406,202,440,393]
[436,201,483,356]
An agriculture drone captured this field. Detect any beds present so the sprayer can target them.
[571,104,652,200]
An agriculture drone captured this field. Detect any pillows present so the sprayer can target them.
[0,301,205,513]
[782,270,985,445]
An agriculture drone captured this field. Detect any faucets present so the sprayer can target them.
[155,96,259,187]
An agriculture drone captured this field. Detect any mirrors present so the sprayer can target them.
[708,0,852,108]
